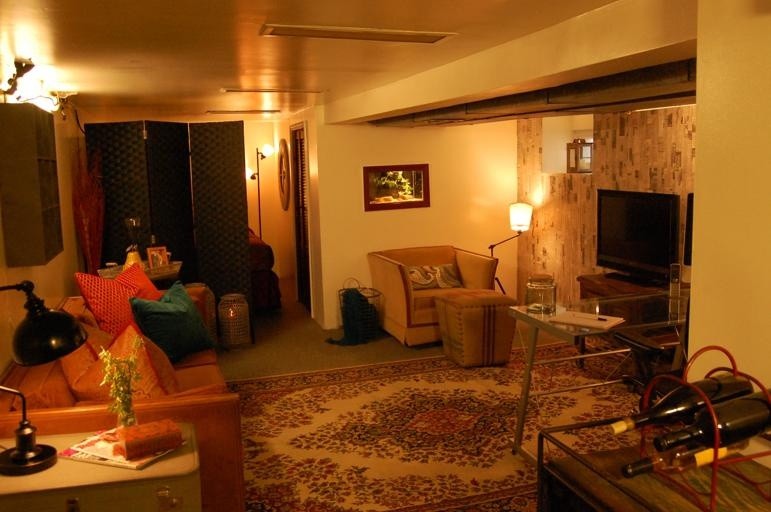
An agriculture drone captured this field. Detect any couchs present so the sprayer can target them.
[1,281,243,511]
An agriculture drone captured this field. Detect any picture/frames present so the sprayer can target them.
[146,246,169,269]
[363,163,430,211]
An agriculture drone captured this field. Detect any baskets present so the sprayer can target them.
[338,277,383,342]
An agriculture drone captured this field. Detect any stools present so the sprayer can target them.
[435,290,516,368]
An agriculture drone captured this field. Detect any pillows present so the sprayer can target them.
[59,264,215,405]
[407,263,461,289]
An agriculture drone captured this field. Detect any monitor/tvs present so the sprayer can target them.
[596,188,680,288]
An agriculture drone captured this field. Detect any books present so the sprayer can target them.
[57,417,188,470]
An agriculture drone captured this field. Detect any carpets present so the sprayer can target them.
[226,337,692,512]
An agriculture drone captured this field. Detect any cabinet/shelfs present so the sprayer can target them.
[1,105,64,269]
[538,411,770,511]
[576,273,690,369]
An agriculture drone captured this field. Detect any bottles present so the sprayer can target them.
[526,274,556,314]
[150,234,158,247]
[608,372,771,478]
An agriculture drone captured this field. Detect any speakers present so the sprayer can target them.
[683,193,693,266]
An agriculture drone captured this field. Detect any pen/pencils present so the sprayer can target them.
[575,315,607,321]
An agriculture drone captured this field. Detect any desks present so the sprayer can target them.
[0,423,203,512]
[509,288,690,476]
[98,260,183,282]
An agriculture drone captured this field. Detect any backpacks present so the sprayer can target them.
[328,287,379,346]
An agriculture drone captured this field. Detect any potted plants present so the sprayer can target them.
[373,170,413,199]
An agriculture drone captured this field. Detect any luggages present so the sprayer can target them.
[251,271,281,315]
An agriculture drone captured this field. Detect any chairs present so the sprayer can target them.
[369,245,497,348]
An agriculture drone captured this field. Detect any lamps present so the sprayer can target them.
[1,56,34,100]
[0,281,83,474]
[490,203,533,295]
[244,143,274,240]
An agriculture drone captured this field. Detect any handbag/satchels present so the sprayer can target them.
[247,227,275,272]
